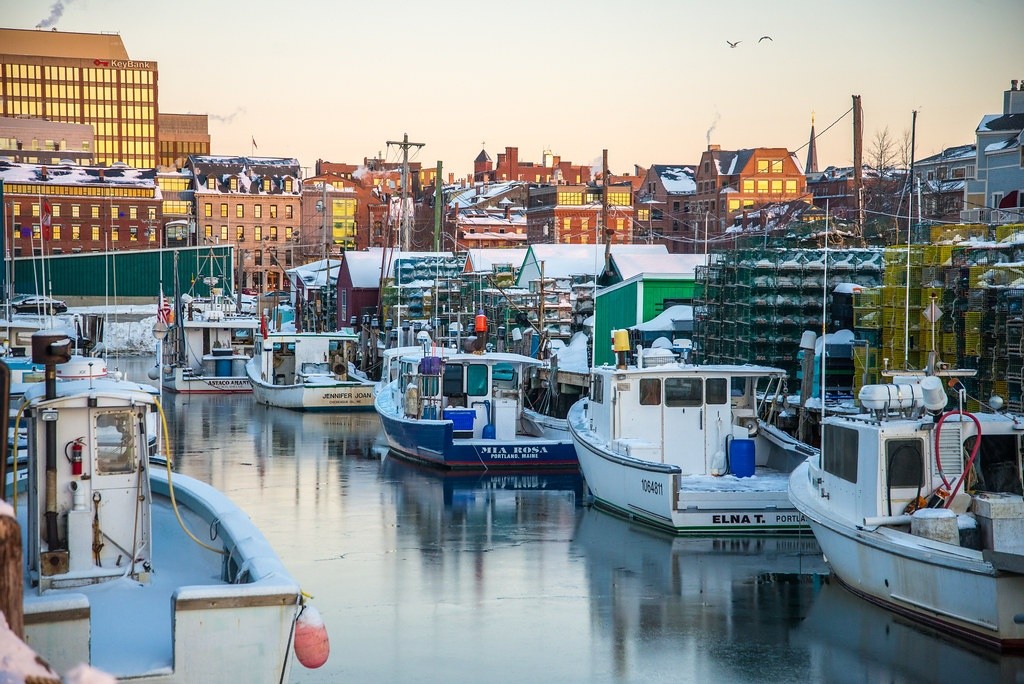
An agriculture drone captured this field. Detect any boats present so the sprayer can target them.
[0,233,169,456]
[0,213,116,356]
[567,211,823,536]
[2,332,329,683]
[787,193,1022,656]
[245,272,377,413]
[375,238,577,469]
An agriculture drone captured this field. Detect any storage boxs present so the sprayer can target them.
[441,409,477,429]
[212,348,233,356]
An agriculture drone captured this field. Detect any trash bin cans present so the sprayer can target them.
[442,408,477,431]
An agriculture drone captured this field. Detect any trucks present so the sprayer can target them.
[183,295,237,314]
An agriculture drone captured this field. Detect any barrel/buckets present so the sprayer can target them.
[729,439,756,478]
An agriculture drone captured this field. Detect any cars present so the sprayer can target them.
[11,296,68,316]
[236,287,258,295]
[253,289,291,301]
[241,296,257,304]
[10,293,39,302]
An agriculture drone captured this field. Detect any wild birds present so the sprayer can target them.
[726,40,742,48]
[758,36,774,43]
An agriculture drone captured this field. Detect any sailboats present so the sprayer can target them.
[146,248,253,394]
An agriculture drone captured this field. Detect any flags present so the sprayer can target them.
[261,315,267,339]
[43,198,51,241]
[157,291,171,323]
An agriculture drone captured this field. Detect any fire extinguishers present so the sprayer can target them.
[72,436,87,475]
